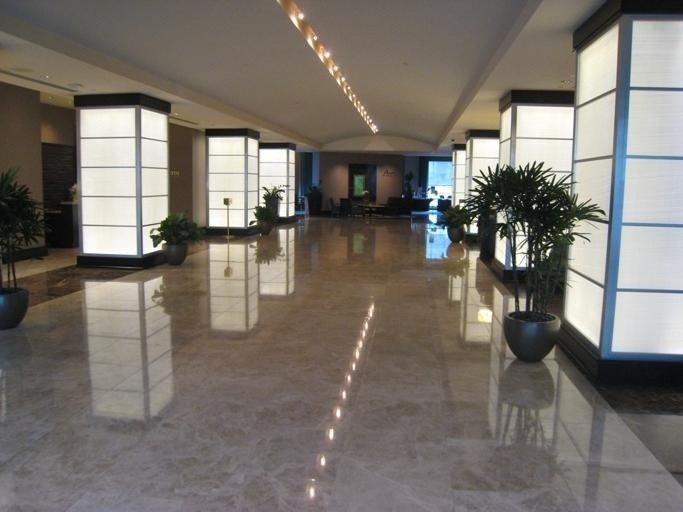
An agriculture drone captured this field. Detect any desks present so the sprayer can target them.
[413,198,433,211]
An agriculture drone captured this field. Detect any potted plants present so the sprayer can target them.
[249,236,285,265]
[466,163,609,361]
[248,185,285,236]
[150,268,210,319]
[306,180,322,217]
[489,361,574,489]
[439,242,470,279]
[0,165,58,330]
[149,211,208,266]
[436,205,474,241]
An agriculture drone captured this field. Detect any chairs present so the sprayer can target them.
[327,193,413,219]
